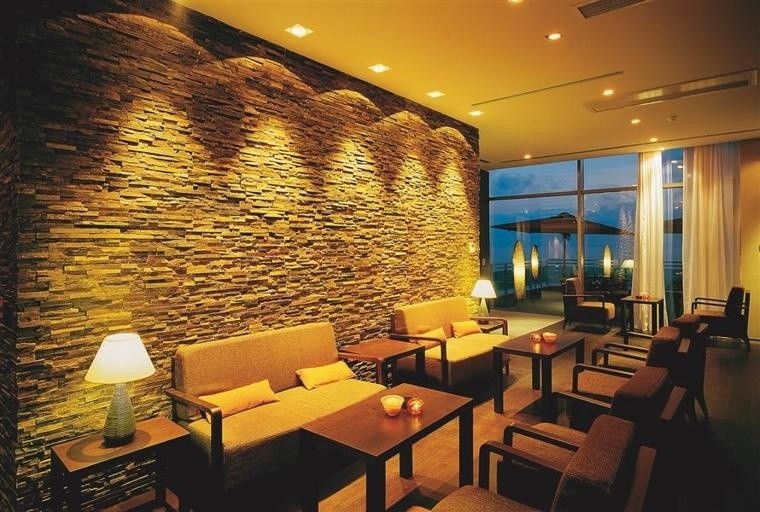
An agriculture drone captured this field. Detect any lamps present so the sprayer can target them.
[530,244,540,281]
[512,241,526,302]
[83,332,157,440]
[470,279,499,322]
[603,245,613,280]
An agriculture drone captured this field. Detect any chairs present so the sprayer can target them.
[690,286,752,351]
[410,416,658,511]
[560,277,617,330]
[497,364,673,511]
[564,326,686,436]
[598,314,711,424]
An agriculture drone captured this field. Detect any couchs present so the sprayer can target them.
[168,321,388,482]
[389,296,511,388]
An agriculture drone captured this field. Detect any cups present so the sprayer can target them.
[636,293,650,301]
[407,399,425,416]
[529,332,542,344]
[382,394,405,418]
[543,332,558,343]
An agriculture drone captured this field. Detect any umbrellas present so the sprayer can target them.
[489,210,635,280]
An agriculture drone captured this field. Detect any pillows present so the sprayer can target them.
[195,379,280,420]
[414,326,447,349]
[293,360,359,388]
[450,319,483,338]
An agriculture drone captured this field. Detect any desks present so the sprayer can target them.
[473,320,504,334]
[618,295,665,336]
[492,329,585,412]
[49,414,194,510]
[298,379,475,512]
[336,338,426,387]
